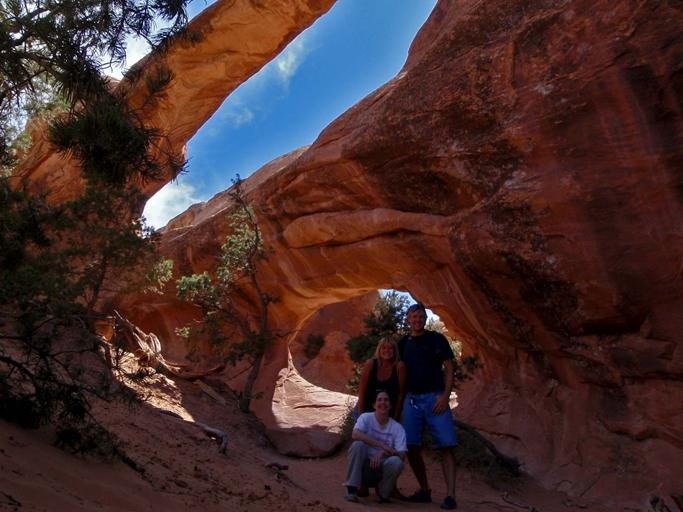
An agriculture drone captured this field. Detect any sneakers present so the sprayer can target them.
[346,493,357,502]
[440,496,457,510]
[406,487,432,503]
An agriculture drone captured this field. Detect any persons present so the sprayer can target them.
[352,335,410,503]
[341,387,409,505]
[394,303,460,511]
